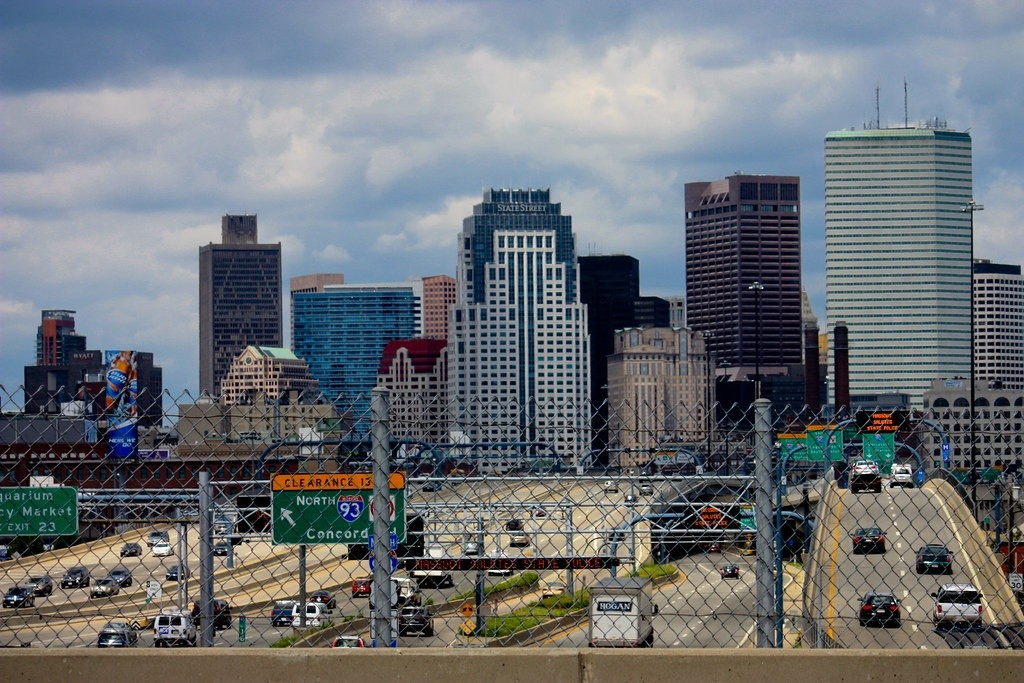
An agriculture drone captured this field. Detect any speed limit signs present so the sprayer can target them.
[1008,573,1023,591]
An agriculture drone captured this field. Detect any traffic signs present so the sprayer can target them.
[862,432,896,461]
[270,472,409,545]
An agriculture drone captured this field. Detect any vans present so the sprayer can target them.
[153,611,197,648]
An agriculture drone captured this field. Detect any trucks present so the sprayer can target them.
[585,578,659,648]
[846,468,883,493]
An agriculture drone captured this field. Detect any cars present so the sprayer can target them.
[147,530,174,556]
[90,578,121,600]
[213,541,229,555]
[704,542,722,553]
[890,467,913,489]
[106,570,133,587]
[190,599,234,630]
[854,461,878,475]
[604,480,654,507]
[856,593,902,628]
[2,586,35,608]
[96,620,138,647]
[849,527,888,554]
[720,564,740,580]
[271,590,337,629]
[120,543,142,556]
[392,472,546,638]
[166,565,190,580]
[352,578,372,597]
[914,543,954,574]
[608,529,625,542]
[24,576,53,596]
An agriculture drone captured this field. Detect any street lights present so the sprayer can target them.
[748,279,769,395]
[960,198,985,528]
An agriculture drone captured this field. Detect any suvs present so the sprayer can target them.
[931,583,984,629]
[59,566,90,588]
